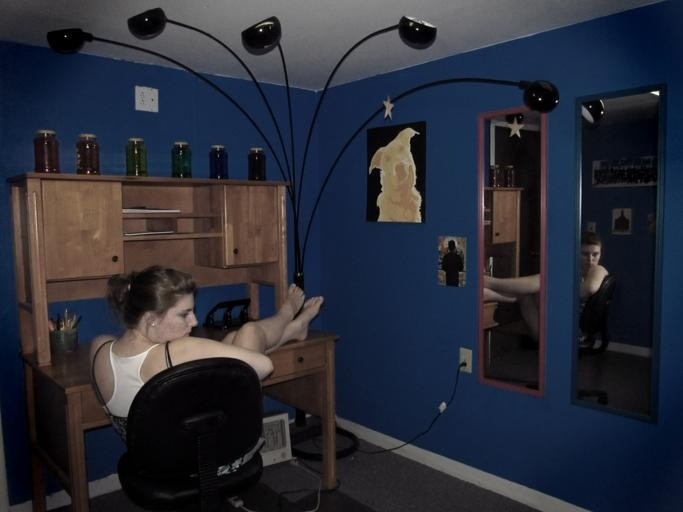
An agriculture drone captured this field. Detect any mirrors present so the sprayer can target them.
[477,105,547,398]
[570,84,667,425]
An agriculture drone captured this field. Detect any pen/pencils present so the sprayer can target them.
[48,304,83,331]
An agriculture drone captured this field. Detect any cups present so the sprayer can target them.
[51,328,78,353]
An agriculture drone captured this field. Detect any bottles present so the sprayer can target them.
[246,146,267,181]
[124,137,148,177]
[506,164,514,187]
[207,145,229,179]
[488,164,502,189]
[73,131,101,175]
[170,140,193,179]
[31,128,60,172]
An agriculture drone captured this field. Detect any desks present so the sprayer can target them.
[484,302,520,328]
[18,319,342,512]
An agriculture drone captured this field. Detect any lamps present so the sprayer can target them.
[581,99,605,124]
[47,8,559,461]
[506,113,524,124]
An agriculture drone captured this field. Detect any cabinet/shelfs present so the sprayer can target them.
[483,186,524,278]
[6,172,291,367]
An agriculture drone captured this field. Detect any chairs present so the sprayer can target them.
[574,274,617,405]
[118,358,266,512]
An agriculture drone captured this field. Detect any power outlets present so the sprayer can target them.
[134,85,159,112]
[459,347,472,373]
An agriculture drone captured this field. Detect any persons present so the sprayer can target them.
[484,232,610,346]
[441,239,463,285]
[89,268,324,448]
